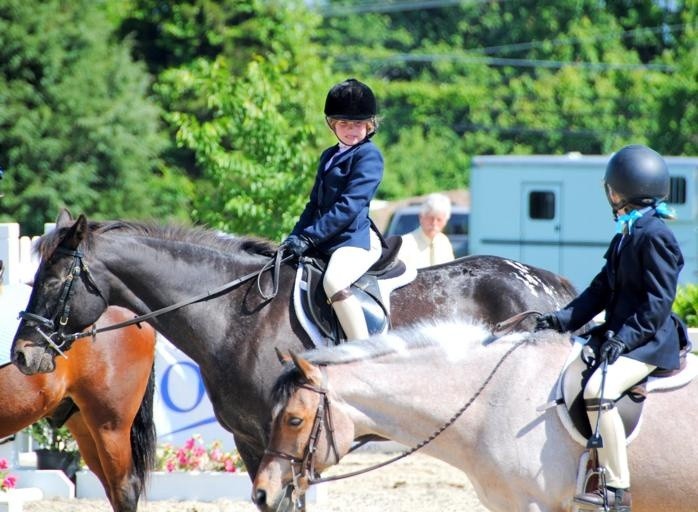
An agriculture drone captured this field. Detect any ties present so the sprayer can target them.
[430,243,435,267]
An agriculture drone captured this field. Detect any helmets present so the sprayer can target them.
[605,144,670,198]
[325,78,376,120]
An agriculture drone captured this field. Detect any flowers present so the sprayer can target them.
[154,434,247,472]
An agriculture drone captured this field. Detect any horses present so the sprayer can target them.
[250,313,698,511]
[11,209,578,511]
[0,304,158,512]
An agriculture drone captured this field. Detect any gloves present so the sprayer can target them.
[599,335,626,364]
[534,314,562,333]
[280,234,313,254]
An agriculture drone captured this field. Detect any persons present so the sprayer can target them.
[394,193,455,272]
[531,145,684,511]
[284,77,386,343]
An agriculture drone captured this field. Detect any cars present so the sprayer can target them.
[384,206,467,256]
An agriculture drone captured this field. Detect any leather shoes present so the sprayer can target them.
[574,487,632,507]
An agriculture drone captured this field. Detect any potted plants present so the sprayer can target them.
[30,417,81,480]
